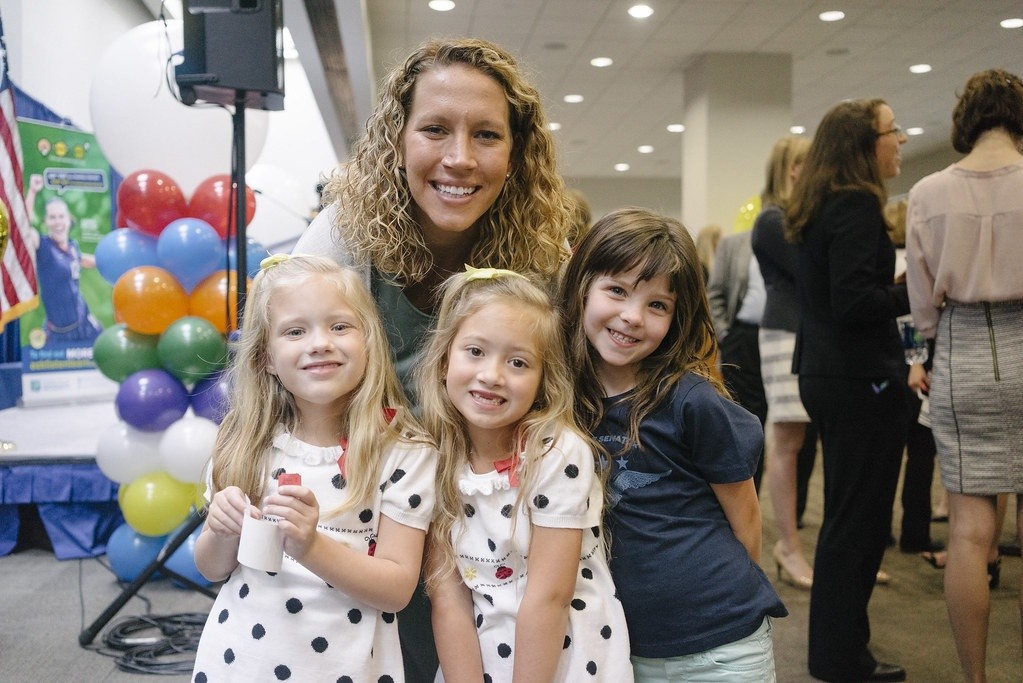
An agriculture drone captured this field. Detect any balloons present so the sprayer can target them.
[217,234,270,279]
[117,171,190,235]
[95,366,243,584]
[112,266,254,336]
[157,319,226,380]
[157,217,222,294]
[190,175,257,239]
[93,321,158,385]
[93,225,161,286]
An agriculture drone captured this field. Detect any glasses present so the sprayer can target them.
[874,125,901,138]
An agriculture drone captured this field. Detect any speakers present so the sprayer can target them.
[175,0,286,112]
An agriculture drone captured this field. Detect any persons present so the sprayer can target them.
[24,175,104,348]
[557,204,790,683]
[750,135,819,587]
[289,34,593,682]
[412,266,637,682]
[784,96,922,683]
[194,258,438,683]
[694,212,817,534]
[882,195,1022,587]
[900,71,1023,682]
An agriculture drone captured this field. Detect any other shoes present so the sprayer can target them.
[930,511,948,520]
[900,541,945,550]
[864,663,906,681]
[999,542,1021,556]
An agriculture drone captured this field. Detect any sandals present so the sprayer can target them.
[922,551,1002,589]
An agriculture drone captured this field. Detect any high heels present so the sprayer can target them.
[875,570,893,582]
[772,540,813,588]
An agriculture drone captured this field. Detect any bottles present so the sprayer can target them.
[902,324,929,365]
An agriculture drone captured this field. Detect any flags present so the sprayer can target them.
[0,11,44,333]
